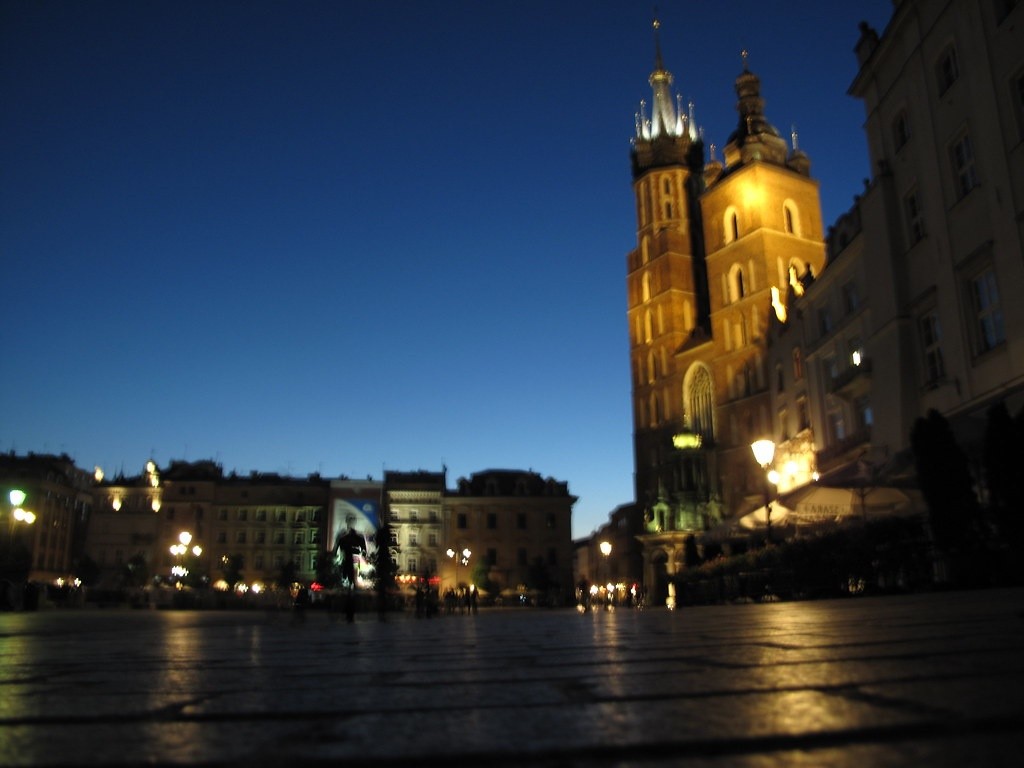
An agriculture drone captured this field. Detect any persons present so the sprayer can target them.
[578,575,641,610]
[412,583,481,617]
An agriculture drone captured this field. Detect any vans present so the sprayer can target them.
[156,589,176,609]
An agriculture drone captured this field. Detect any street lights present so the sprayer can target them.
[751,433,780,599]
[170,529,202,589]
[446,546,471,585]
[600,541,612,608]
